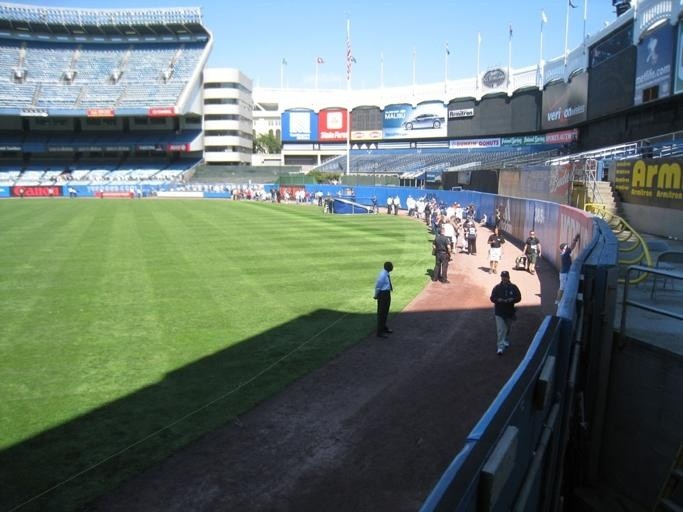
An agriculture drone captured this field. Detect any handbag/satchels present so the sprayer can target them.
[431,247,435,256]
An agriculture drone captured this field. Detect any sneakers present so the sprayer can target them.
[496,342,508,355]
[554,300,560,304]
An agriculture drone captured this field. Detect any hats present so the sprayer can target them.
[499,271,509,277]
[559,243,568,249]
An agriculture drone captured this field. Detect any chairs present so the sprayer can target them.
[637,238,683,304]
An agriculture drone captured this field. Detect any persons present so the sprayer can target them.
[490,272,522,355]
[554,232,580,305]
[373,261,394,339]
[521,230,541,275]
[370,194,506,284]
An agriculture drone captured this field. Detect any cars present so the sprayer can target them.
[400,114,440,130]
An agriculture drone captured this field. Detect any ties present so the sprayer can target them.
[387,275,392,291]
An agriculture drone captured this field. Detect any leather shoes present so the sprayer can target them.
[382,329,392,339]
